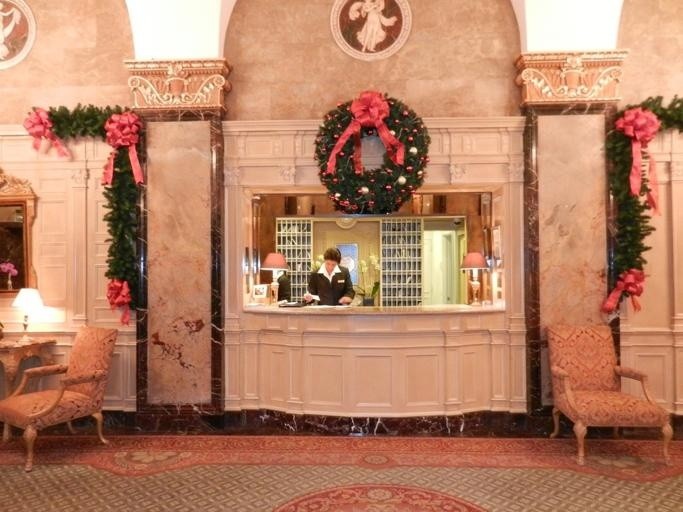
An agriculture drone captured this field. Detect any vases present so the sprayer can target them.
[362,297,374,305]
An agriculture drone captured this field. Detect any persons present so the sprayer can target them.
[302,247,355,306]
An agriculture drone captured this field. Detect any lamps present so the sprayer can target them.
[460,252,489,307]
[260,253,288,308]
[11,287,44,342]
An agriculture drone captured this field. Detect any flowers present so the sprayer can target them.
[314,92,432,216]
[354,253,381,298]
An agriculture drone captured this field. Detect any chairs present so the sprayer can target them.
[547,322,673,467]
[0,325,119,474]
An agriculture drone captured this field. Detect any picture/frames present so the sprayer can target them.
[0,167,38,301]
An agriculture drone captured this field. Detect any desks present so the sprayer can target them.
[0,335,56,443]
